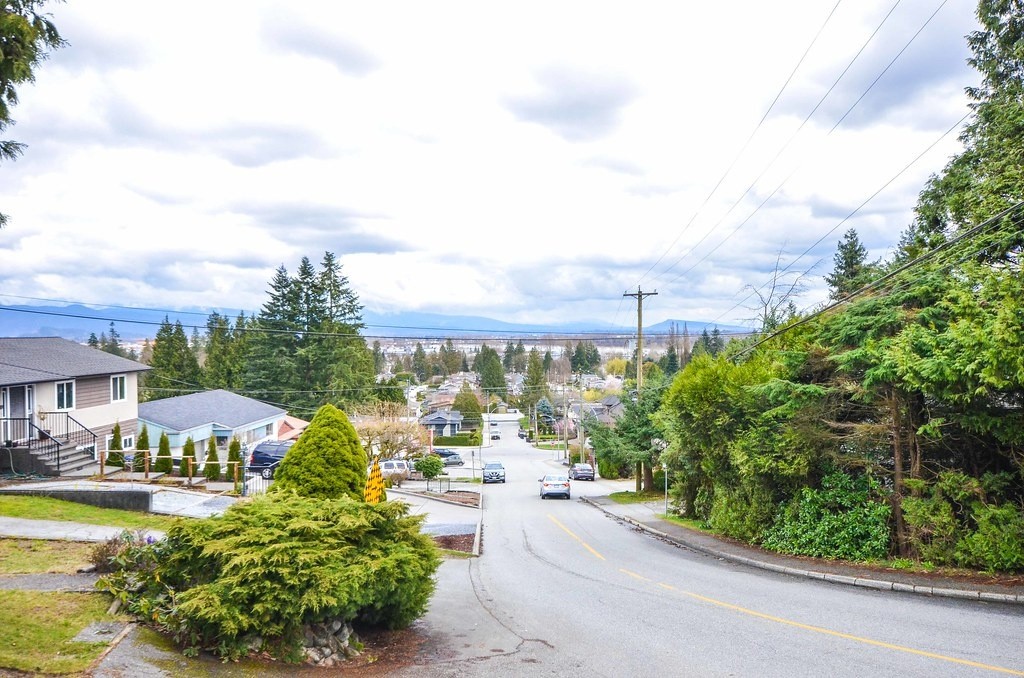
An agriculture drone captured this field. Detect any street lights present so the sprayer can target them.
[488,404,506,446]
[569,399,598,422]
[540,414,560,460]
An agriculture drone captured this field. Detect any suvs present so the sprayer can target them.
[481,461,506,484]
[248,440,297,480]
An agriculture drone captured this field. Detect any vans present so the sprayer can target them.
[368,460,411,481]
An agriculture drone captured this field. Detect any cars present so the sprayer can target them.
[490,419,497,426]
[442,454,465,467]
[518,429,528,437]
[568,462,595,481]
[538,475,571,499]
[431,448,457,459]
[410,458,422,469]
[491,429,501,441]
